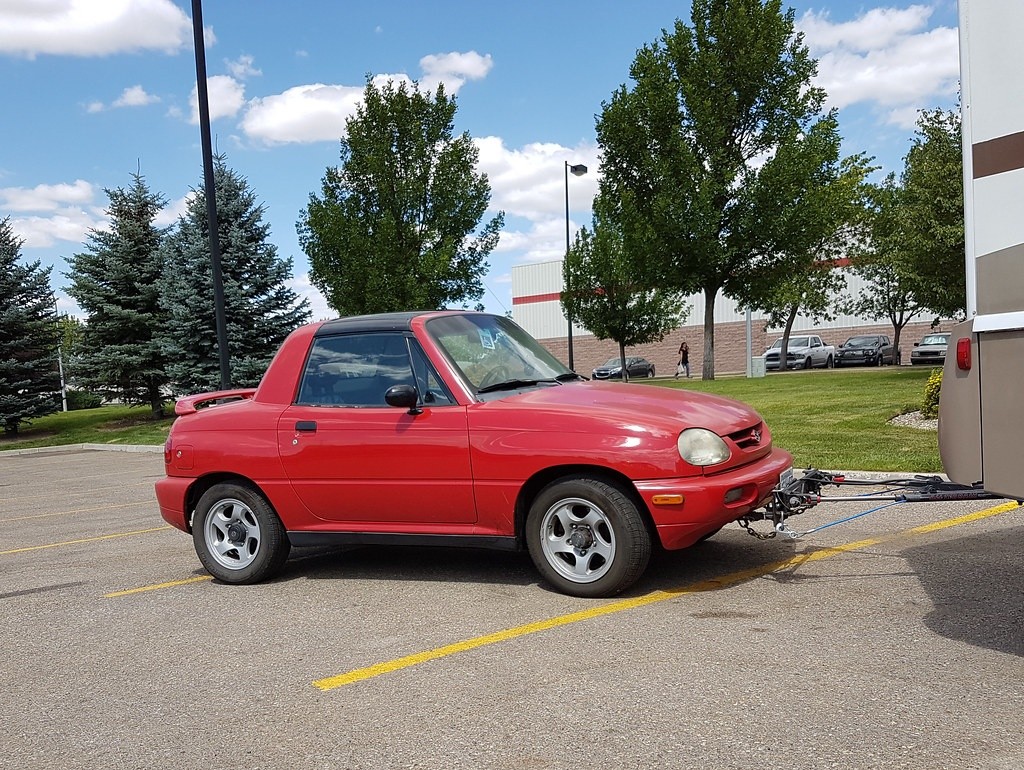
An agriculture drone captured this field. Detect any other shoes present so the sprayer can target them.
[675,375,678,380]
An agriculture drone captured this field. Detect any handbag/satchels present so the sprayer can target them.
[676,362,684,375]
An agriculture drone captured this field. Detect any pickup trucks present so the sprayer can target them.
[762,335,835,370]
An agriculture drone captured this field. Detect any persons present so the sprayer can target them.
[674,342,693,380]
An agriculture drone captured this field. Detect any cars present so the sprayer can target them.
[565,160,588,372]
[910,333,952,365]
[834,334,901,368]
[592,356,656,380]
[154,310,794,599]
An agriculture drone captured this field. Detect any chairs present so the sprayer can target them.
[377,353,429,396]
[304,363,345,405]
